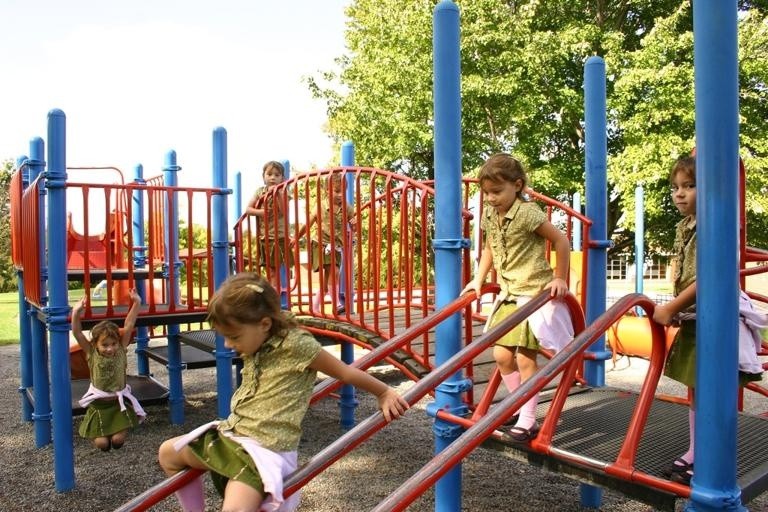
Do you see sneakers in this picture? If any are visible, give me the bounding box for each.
[332,304,346,315]
[501,413,540,443]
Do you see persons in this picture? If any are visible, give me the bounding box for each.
[158,272,410,512]
[459,153,574,444]
[246,160,295,290]
[651,155,767,484]
[71,287,147,451]
[289,173,357,315]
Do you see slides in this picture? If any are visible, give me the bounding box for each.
[67,209,184,380]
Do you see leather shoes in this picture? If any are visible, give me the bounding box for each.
[672,456,694,485]
